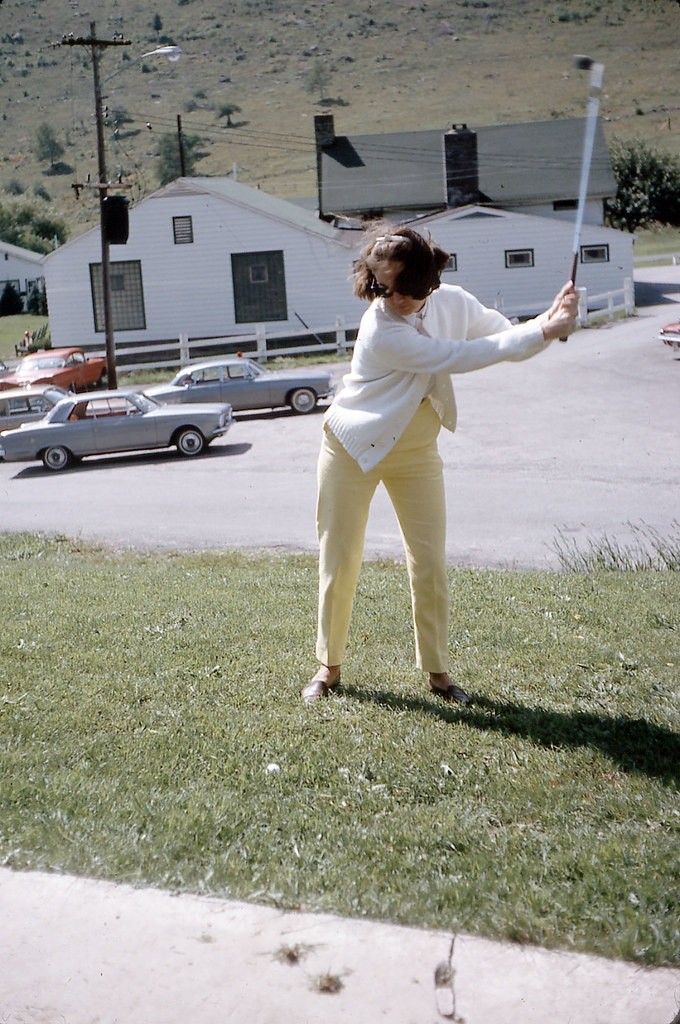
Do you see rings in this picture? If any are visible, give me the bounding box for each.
[574,311,579,317]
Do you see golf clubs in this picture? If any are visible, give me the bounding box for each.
[558,52,608,343]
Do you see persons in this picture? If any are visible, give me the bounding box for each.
[23,331,33,348]
[301,228,581,702]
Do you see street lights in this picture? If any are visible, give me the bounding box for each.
[93,43,185,391]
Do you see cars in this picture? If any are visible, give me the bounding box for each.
[0,390,236,472]
[140,357,334,415]
[0,346,111,393]
[0,382,73,428]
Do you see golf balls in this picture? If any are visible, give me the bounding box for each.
[265,763,282,777]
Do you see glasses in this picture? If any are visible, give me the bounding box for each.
[371,277,413,298]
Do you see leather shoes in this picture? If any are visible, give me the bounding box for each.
[300,672,341,703]
[428,675,471,705]
